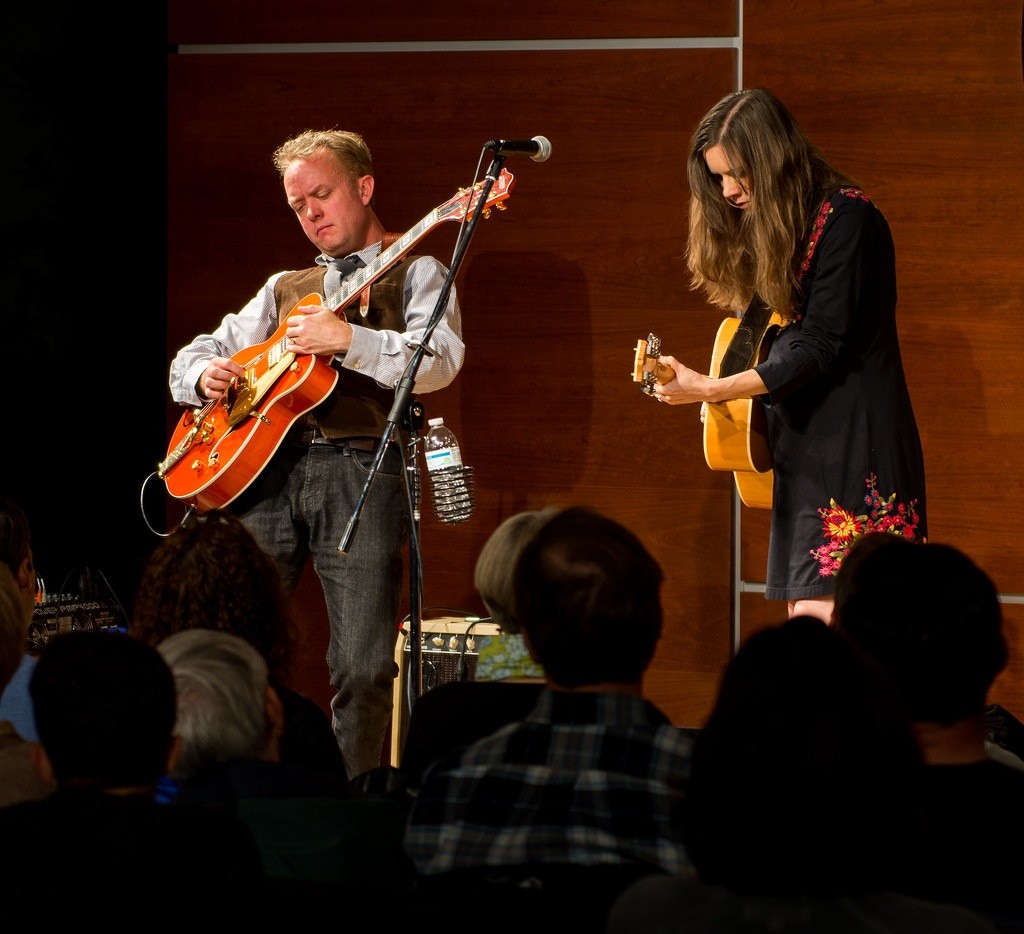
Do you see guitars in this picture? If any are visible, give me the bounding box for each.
[162,163,517,512]
[628,311,795,510]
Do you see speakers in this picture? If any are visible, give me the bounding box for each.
[386,617,505,768]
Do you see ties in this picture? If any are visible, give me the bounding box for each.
[323,254,368,299]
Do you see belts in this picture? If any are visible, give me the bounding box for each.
[281,423,375,452]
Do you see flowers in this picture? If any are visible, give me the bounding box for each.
[808,471,928,578]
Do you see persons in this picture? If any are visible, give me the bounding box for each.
[831,531,1024,933]
[610,612,995,934]
[385,506,687,931]
[165,131,467,779]
[639,88,926,626]
[0,494,349,934]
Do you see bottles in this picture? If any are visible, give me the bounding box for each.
[424,417,472,525]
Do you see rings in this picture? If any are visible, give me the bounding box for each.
[293,338,296,344]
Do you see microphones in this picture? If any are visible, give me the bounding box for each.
[484,135,551,162]
